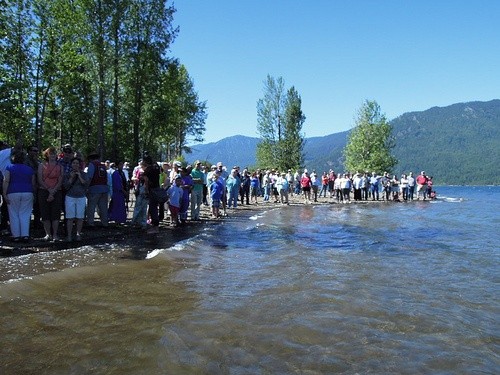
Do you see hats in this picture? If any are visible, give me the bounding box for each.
[194,160,201,166]
[88,150,99,157]
[173,161,181,168]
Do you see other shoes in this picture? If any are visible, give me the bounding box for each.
[13,234,82,243]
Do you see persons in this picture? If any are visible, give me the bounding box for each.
[63,157,92,242]
[37,146,64,242]
[3,150,38,240]
[0,140,442,233]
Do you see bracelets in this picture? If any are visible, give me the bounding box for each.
[3,192,6,195]
[46,186,49,190]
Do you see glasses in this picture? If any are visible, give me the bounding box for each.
[31,149,39,153]
[64,150,71,153]
